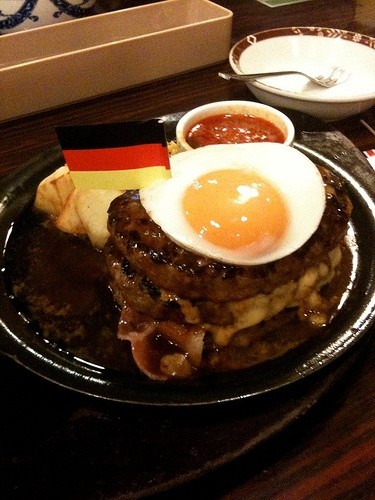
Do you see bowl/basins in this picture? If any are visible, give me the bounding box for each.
[176,101,294,151]
[229,26,375,121]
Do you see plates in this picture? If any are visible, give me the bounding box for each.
[0,113,375,406]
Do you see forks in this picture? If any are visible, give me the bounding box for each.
[218,65,349,87]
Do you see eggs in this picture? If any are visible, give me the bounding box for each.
[140,144,325,263]
